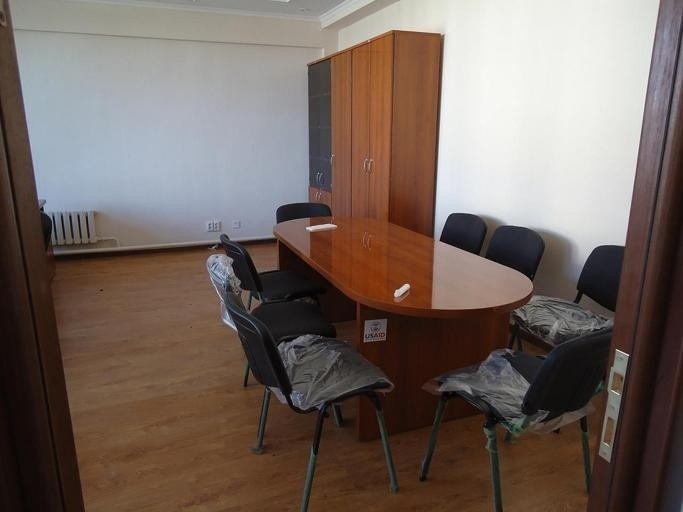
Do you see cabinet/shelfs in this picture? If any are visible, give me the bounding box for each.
[309,29,442,239]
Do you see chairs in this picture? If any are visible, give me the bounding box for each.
[439,212,488,257]
[511,243,624,352]
[275,203,333,223]
[419,325,615,512]
[224,291,401,512]
[206,246,337,392]
[218,232,321,312]
[485,225,546,349]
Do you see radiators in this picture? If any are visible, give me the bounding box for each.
[44,208,98,246]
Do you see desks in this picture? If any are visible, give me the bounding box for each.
[272,216,537,444]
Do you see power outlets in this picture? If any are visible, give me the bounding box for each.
[232,219,240,229]
[208,220,213,231]
[214,221,220,231]
